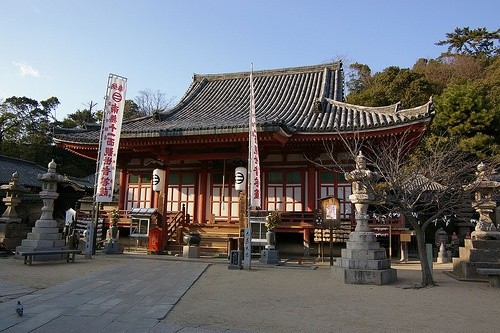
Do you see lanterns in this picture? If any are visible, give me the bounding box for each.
[152,168,166,191]
[235,167,247,191]
